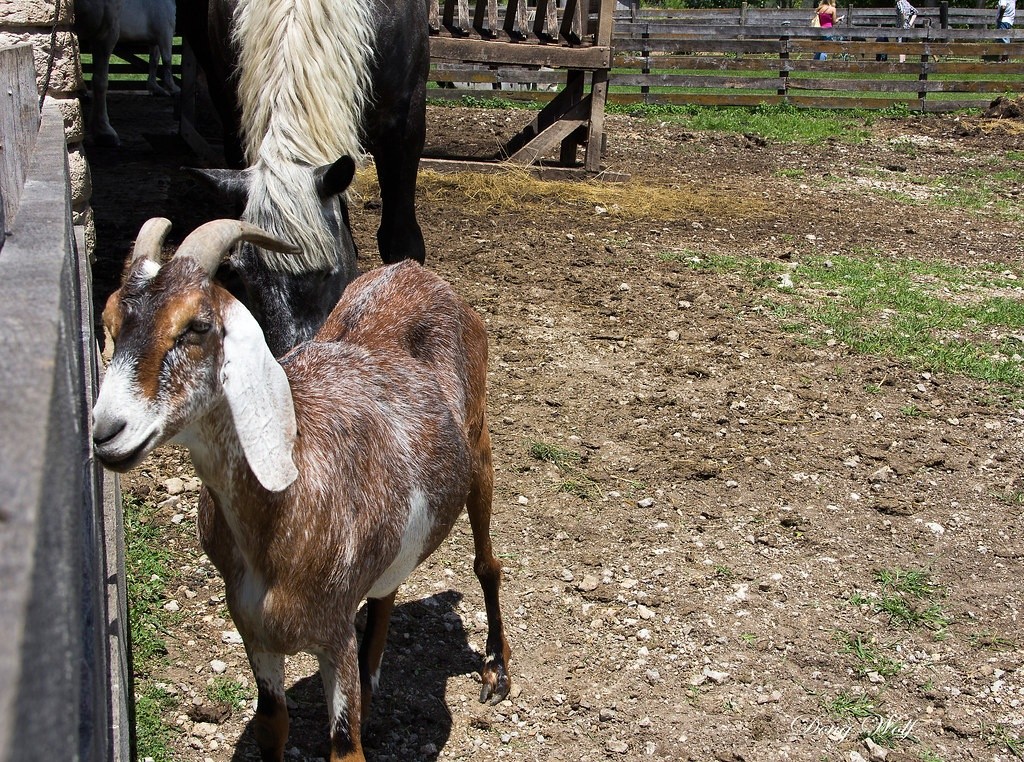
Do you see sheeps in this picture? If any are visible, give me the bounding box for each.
[88,215,514,762]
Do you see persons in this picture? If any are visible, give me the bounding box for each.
[996,0,1015,61]
[895,0,918,63]
[813,0,844,61]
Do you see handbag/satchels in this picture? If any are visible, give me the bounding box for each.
[808,11,821,27]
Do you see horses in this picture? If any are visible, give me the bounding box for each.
[71,0,431,359]
